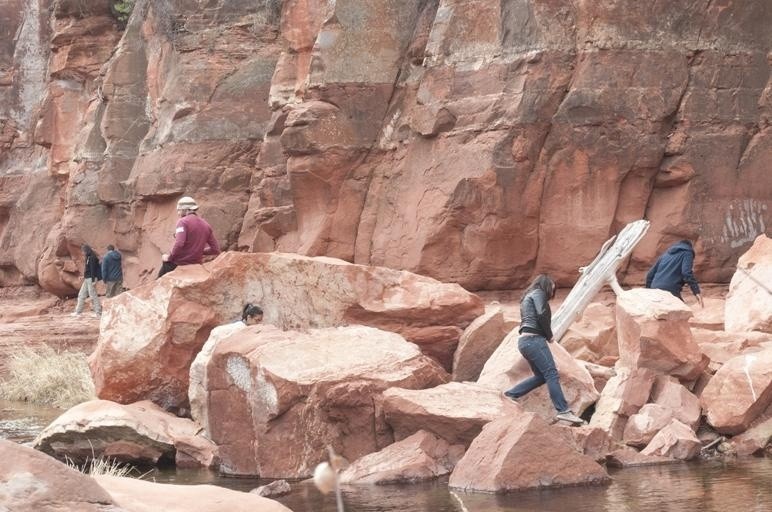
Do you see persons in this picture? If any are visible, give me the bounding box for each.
[233,301,264,326]
[73,245,104,316]
[100,244,123,297]
[646,240,704,309]
[158,197,222,279]
[505,272,585,424]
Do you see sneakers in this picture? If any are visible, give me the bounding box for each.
[557,412,583,423]
[503,392,521,405]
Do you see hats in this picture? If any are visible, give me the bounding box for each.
[177,197,198,210]
